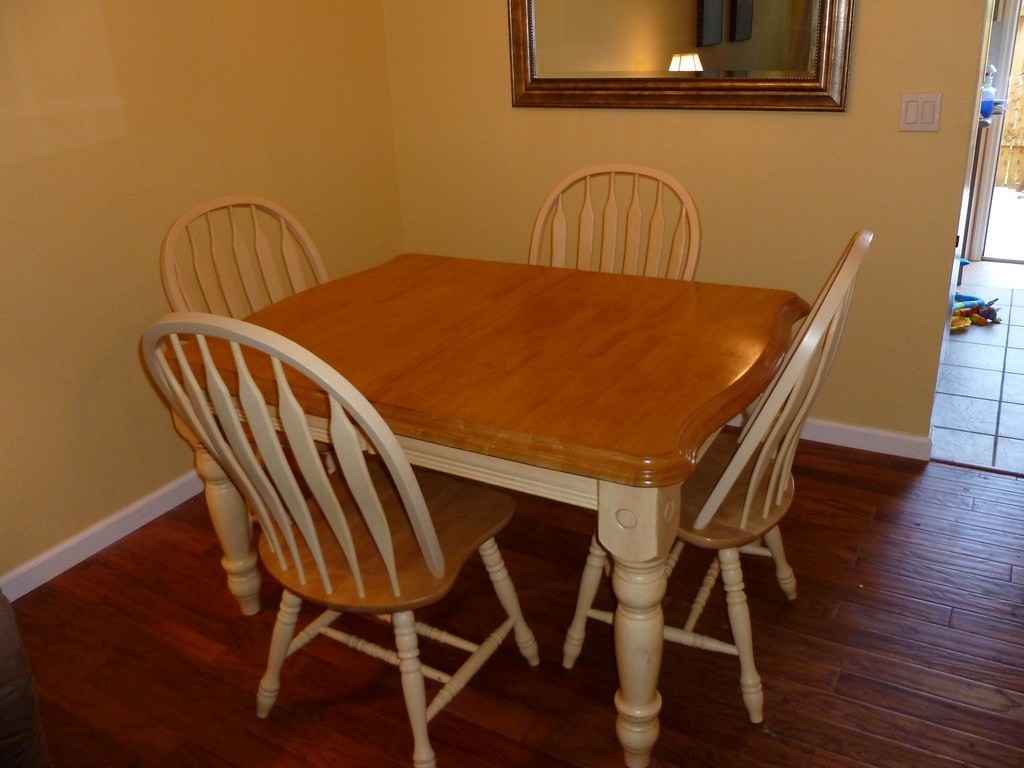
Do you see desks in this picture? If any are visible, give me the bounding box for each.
[154,254,813,768]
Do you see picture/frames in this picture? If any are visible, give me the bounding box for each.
[730,0,753,41]
[697,0,724,46]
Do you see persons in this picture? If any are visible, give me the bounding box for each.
[0,589,54,768]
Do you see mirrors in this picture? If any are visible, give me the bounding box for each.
[507,0,855,111]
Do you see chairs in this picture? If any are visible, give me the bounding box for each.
[560,229,873,725]
[530,164,702,283]
[162,197,336,533]
[139,310,541,768]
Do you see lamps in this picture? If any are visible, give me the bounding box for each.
[669,53,703,72]
